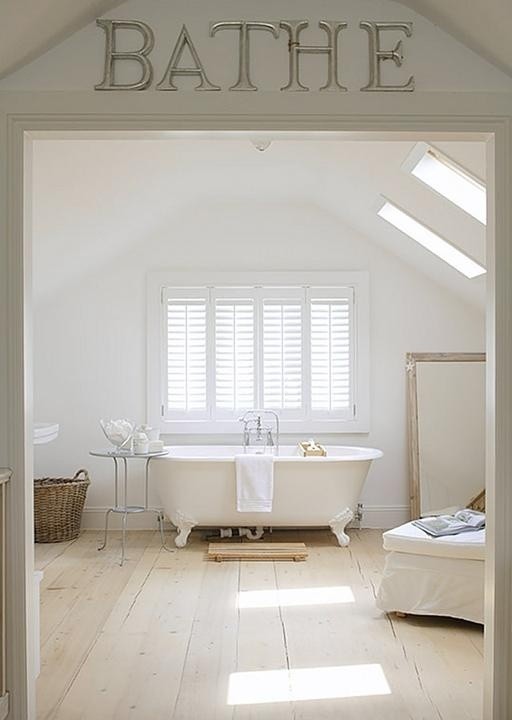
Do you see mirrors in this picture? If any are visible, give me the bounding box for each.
[406,352,486,522]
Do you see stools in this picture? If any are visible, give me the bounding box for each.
[377,516,486,627]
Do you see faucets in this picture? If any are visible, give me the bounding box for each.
[256,434,264,442]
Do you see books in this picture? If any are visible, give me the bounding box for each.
[411,509,486,537]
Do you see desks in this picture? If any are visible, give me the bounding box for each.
[89,448,173,567]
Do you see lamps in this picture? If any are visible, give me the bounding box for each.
[250,138,271,152]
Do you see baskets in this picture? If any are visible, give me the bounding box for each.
[34,469,91,543]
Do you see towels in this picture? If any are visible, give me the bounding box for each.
[235,453,274,513]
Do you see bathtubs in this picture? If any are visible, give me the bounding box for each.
[148,445,385,548]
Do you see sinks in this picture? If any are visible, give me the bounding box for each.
[33,423,60,445]
